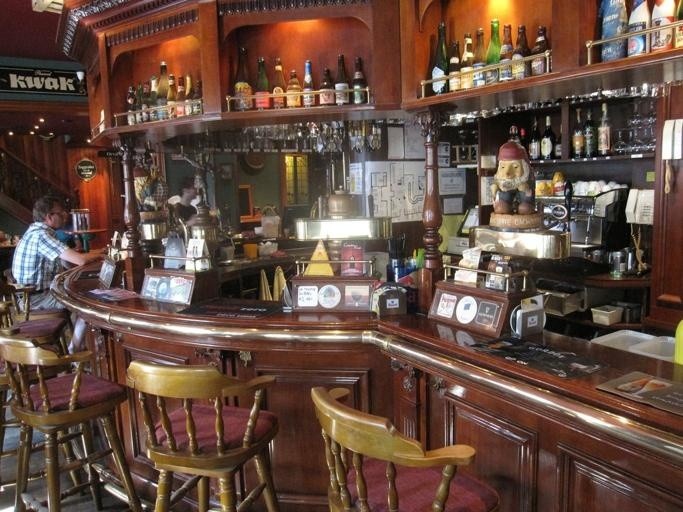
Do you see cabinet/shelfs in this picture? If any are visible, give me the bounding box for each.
[89,0,399,147]
[477,90,657,169]
[546,268,646,334]
[400,1,683,111]
[220,275,258,298]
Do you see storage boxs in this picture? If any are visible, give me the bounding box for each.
[590,330,657,352]
[591,304,624,326]
[629,336,675,361]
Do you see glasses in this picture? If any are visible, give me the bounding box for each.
[49,211,64,216]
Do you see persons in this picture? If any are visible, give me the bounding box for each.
[11,197,107,310]
[492,143,535,214]
[175,175,198,225]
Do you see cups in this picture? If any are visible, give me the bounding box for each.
[218,124,296,150]
[219,246,234,260]
[243,244,258,259]
[611,128,628,154]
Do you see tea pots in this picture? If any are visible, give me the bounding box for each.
[328,185,356,216]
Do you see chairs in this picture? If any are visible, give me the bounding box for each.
[124,358,280,511]
[5,268,17,286]
[312,387,501,510]
[1,336,142,510]
[0,302,67,355]
[0,289,69,355]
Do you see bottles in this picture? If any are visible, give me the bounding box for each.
[627,99,657,151]
[126,60,202,126]
[429,19,550,93]
[232,47,254,110]
[287,70,301,107]
[253,56,272,109]
[334,54,350,104]
[507,102,611,160]
[319,67,335,105]
[271,56,287,108]
[351,56,367,104]
[302,60,315,106]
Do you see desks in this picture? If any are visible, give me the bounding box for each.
[64,229,106,252]
